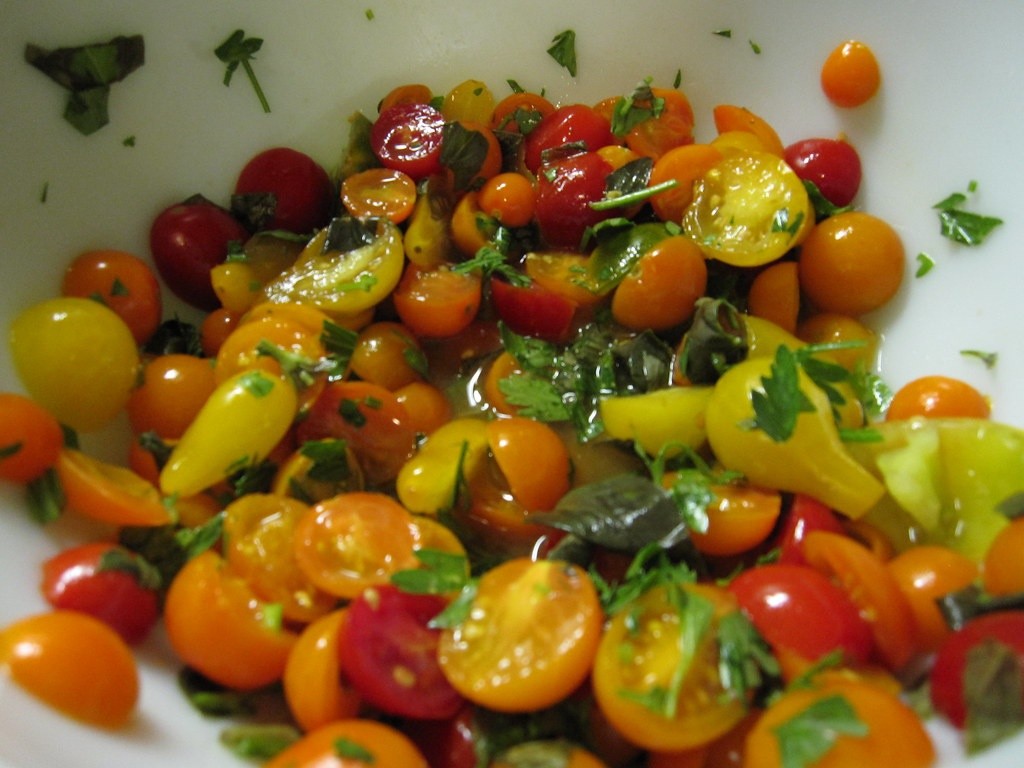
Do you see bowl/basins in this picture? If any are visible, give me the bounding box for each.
[0,0,1024,768]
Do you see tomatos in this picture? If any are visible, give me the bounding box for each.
[0,40,1024,768]
